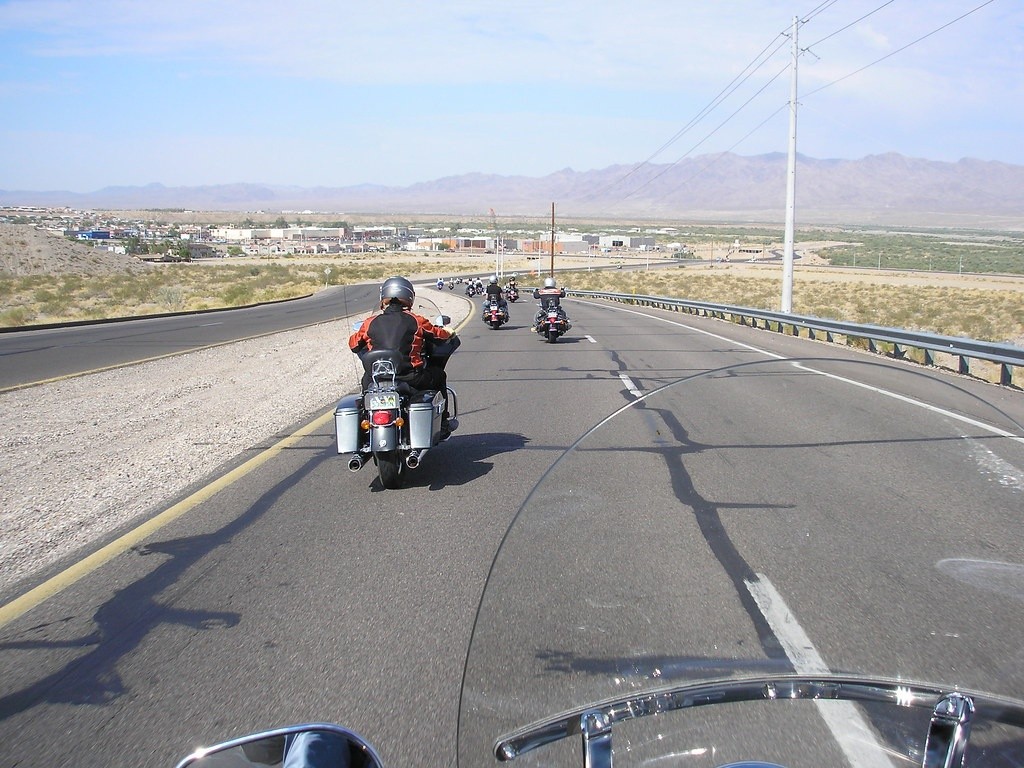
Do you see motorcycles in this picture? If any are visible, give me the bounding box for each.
[474,282,484,295]
[447,281,455,290]
[482,293,508,330]
[533,288,572,344]
[465,283,476,298]
[456,279,462,285]
[437,281,444,291]
[505,285,520,303]
[334,313,459,490]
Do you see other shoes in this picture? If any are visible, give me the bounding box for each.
[566,324,572,330]
[440,419,459,438]
[531,327,538,333]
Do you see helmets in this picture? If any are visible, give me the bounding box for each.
[379,275,416,308]
[489,275,499,284]
[469,277,472,281]
[477,276,480,280]
[544,277,557,288]
[511,277,516,281]
[509,278,513,281]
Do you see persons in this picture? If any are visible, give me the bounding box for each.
[349,276,456,440]
[437,276,570,331]
